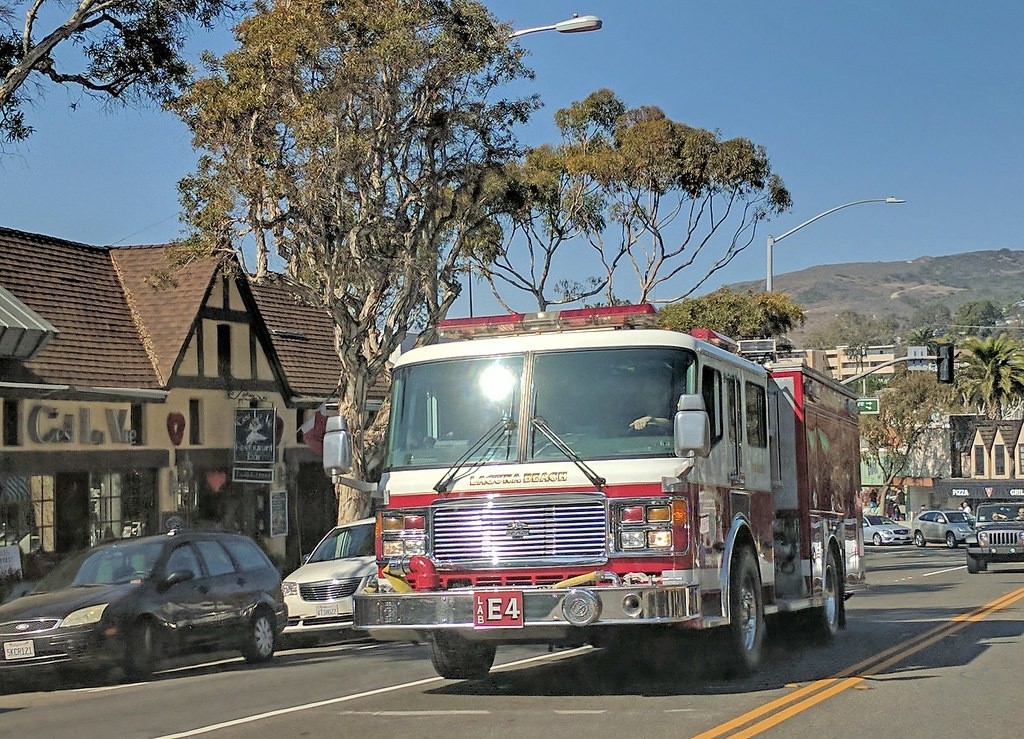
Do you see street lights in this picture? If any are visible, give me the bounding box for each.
[399,14,603,201]
[764,196,906,293]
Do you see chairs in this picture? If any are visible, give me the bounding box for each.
[113,566,137,585]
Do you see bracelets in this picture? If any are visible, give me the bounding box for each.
[652,416,655,422]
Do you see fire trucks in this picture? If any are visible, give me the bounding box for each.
[321,302,866,686]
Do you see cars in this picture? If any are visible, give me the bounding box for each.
[862,514,914,546]
[0,528,290,687]
[279,515,381,647]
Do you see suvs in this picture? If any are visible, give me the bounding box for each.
[967,501,1024,574]
[911,508,977,549]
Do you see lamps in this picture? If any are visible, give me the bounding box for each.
[49,408,60,419]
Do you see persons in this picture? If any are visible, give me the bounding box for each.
[1013,507,1024,520]
[629,382,673,430]
[165,517,182,530]
[991,512,1007,520]
[918,504,927,514]
[959,502,971,521]
[886,498,901,521]
[869,489,878,508]
